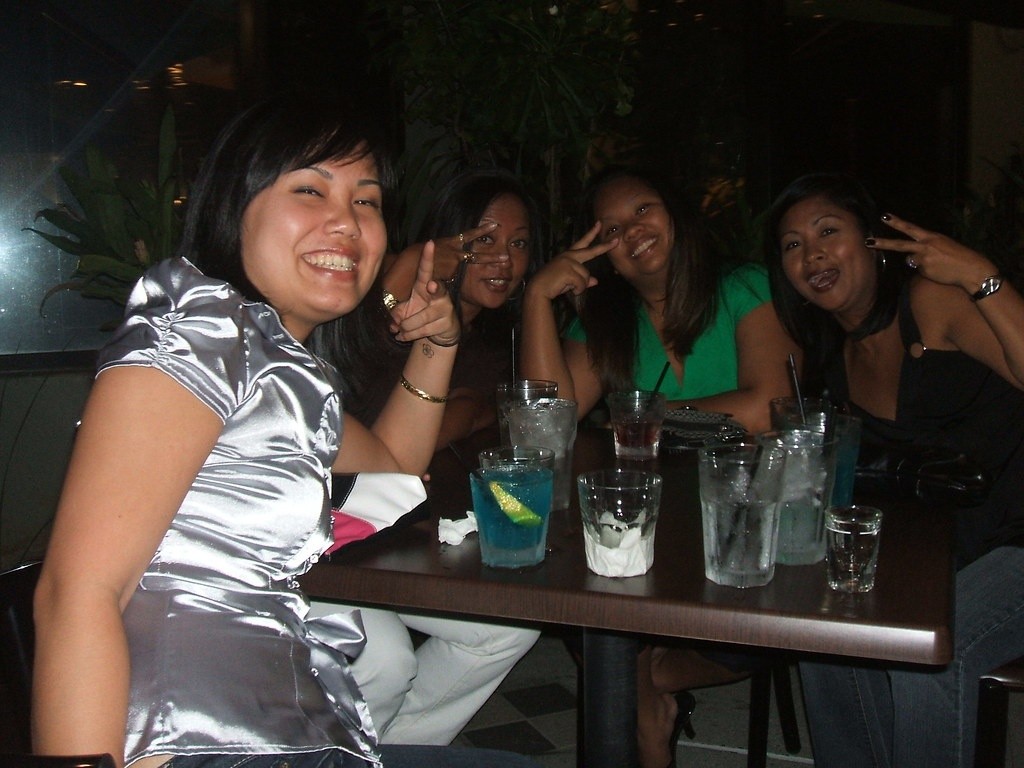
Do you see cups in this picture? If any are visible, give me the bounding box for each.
[479,446,554,474]
[758,432,838,565]
[496,380,558,447]
[470,465,554,569]
[825,506,882,594]
[698,446,788,587]
[828,414,863,507]
[605,391,666,462]
[577,471,662,578]
[509,398,579,511]
[769,397,838,443]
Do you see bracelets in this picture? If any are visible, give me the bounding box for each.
[383,291,398,308]
[428,336,459,347]
[399,373,449,403]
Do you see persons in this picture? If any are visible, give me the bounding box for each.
[28,85,539,768]
[760,172,1024,768]
[520,162,803,768]
[330,168,542,747]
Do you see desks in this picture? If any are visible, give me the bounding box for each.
[297,421,952,767]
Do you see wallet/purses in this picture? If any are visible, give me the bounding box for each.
[660,405,747,449]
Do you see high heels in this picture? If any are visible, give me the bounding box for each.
[639,691,697,768]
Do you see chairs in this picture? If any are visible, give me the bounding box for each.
[0,559,121,768]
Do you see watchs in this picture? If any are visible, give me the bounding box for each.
[970,270,1005,302]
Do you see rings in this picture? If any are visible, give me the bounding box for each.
[906,255,918,268]
[464,249,474,264]
[459,233,464,243]
